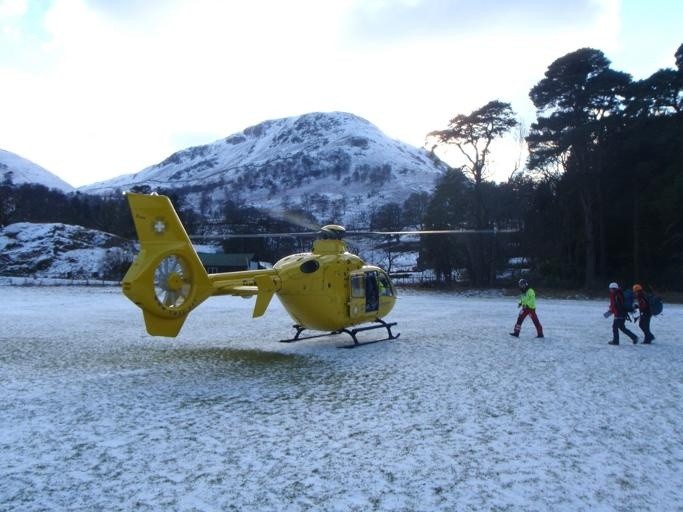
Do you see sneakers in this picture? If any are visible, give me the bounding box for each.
[510,332,519,337]
[535,335,543,338]
[634,336,638,344]
[643,334,654,344]
[609,341,618,345]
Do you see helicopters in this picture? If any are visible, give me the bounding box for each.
[123,188,526,350]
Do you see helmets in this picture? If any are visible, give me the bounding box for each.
[609,283,618,288]
[518,279,529,288]
[633,285,641,293]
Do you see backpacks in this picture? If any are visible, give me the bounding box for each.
[615,290,634,311]
[642,292,662,315]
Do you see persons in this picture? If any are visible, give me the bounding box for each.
[603,282,639,346]
[509,278,544,339]
[633,284,656,345]
